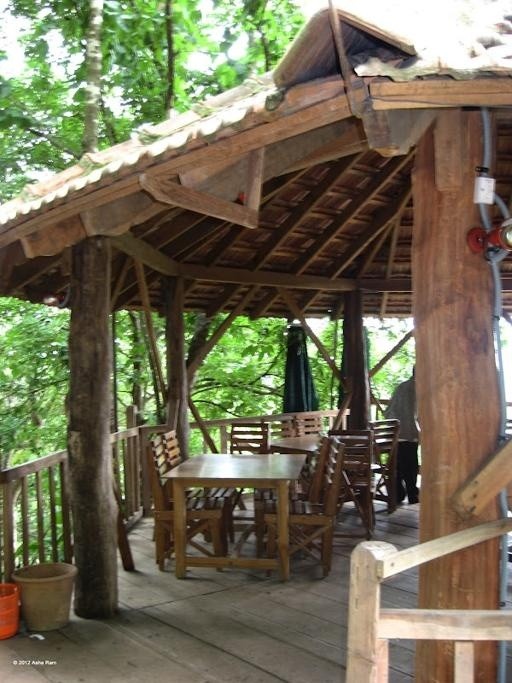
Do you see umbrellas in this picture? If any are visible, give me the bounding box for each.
[279,323,324,437]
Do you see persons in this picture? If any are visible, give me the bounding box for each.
[382,362,419,507]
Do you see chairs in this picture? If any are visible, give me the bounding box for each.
[145,413,402,581]
